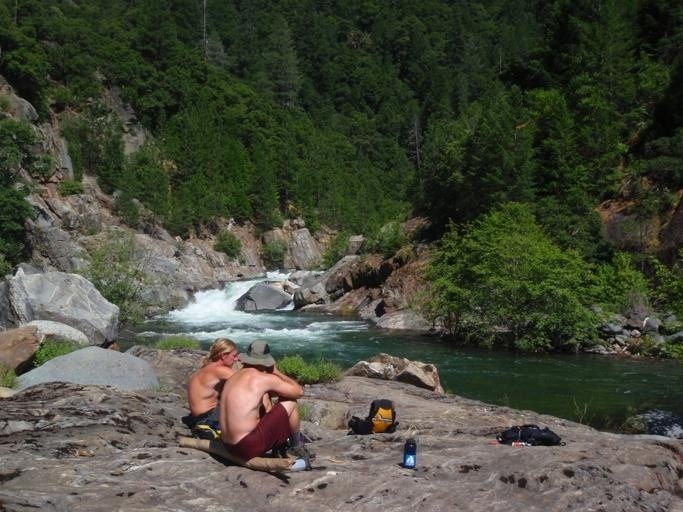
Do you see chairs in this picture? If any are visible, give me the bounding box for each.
[285,445,315,461]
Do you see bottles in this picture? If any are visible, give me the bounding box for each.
[237,340,275,367]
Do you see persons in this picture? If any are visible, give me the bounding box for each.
[218,339,316,464]
[187,339,274,435]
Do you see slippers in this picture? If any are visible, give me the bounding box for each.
[364,399,398,433]
[495,425,565,446]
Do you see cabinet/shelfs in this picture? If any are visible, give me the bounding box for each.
[512,442,531,447]
[386,421,399,433]
[403,438,417,468]
